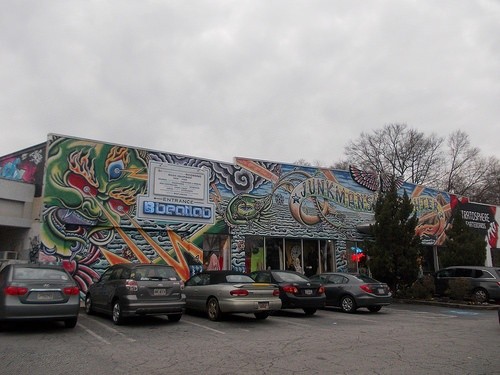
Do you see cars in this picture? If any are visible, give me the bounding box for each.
[0,264,80,329]
[184,270,282,322]
[309,272,392,313]
[249,269,326,315]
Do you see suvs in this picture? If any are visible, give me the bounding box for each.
[84,264,184,325]
[430,265,500,304]
